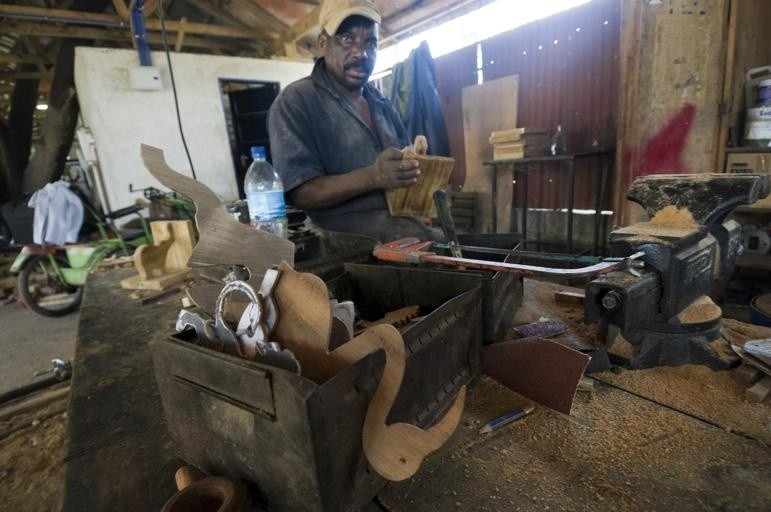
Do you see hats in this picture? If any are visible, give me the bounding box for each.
[319,0,382,39]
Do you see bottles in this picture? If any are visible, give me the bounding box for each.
[549,124,568,156]
[244,146,288,241]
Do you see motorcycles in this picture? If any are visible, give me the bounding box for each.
[7,183,197,316]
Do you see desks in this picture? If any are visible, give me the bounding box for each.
[483,151,609,258]
[61,262,771,511]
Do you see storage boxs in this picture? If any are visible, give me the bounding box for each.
[149,232,525,512]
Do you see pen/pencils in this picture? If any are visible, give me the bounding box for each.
[477,403,536,434]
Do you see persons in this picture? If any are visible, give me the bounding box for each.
[265,0,448,267]
